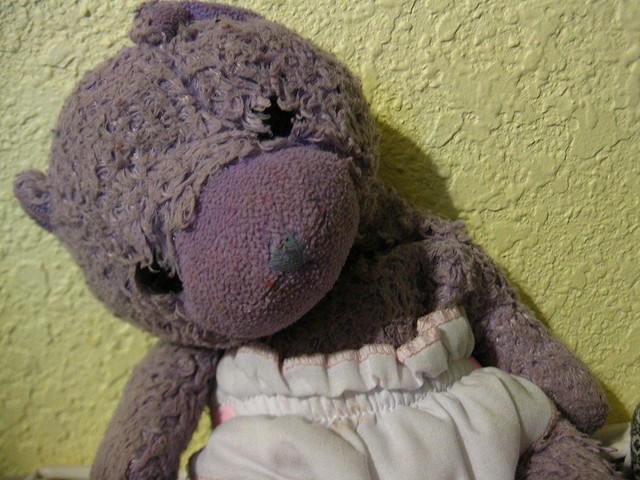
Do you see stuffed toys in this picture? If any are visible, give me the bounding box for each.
[12,1,624,480]
